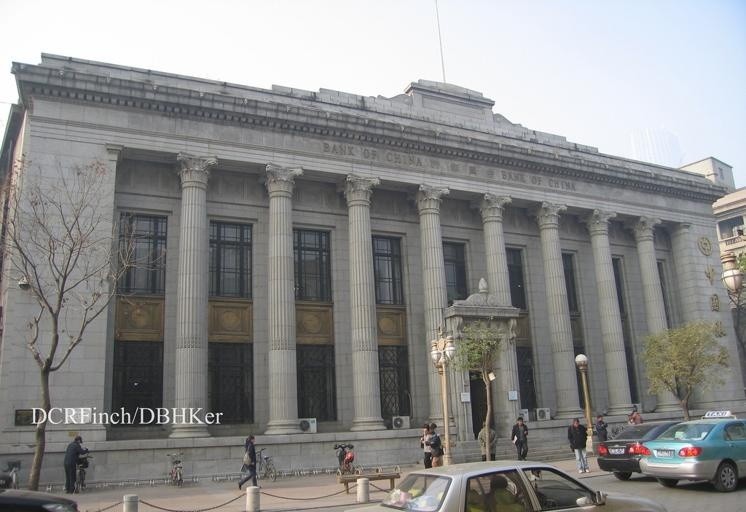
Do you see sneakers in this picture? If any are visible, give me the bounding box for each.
[579,470,583,473]
[585,469,589,473]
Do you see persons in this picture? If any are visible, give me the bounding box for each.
[595,415,608,441]
[510,416,529,461]
[486,475,508,512]
[426,423,444,467]
[237,434,261,490]
[478,420,497,460]
[63,435,88,493]
[420,424,432,468]
[567,418,590,473]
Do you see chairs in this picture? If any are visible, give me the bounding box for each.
[467,488,525,511]
[675,430,708,440]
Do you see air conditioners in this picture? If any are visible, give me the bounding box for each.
[533,407,551,420]
[299,417,317,433]
[392,415,411,429]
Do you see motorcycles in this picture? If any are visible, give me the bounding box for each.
[334,442,354,476]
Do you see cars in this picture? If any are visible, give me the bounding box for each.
[638,410,746,492]
[0,483,79,512]
[596,419,681,481]
[342,459,669,512]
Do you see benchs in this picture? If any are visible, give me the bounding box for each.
[337,472,400,494]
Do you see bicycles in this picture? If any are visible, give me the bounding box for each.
[4,459,22,488]
[74,453,94,492]
[254,447,277,481]
[168,451,185,486]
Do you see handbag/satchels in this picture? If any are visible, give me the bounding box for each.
[243,450,254,467]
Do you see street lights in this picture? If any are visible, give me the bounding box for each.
[721,249,746,293]
[430,325,459,465]
[575,353,595,429]
